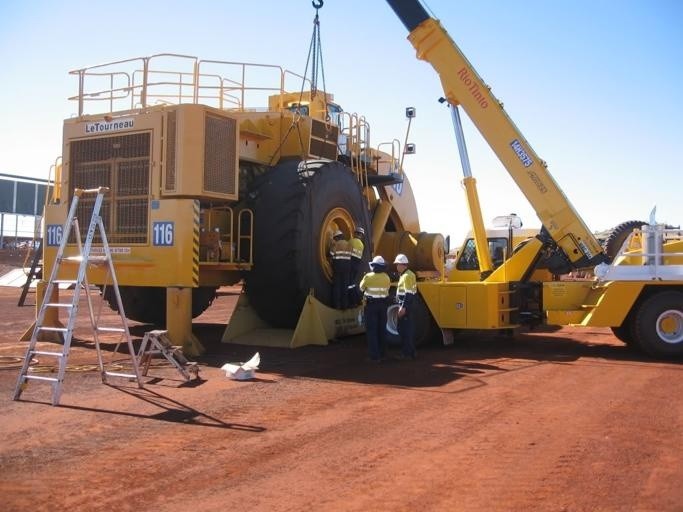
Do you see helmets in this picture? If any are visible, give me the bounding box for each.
[394,254,408,264]
[354,227,364,234]
[333,231,344,238]
[370,255,386,266]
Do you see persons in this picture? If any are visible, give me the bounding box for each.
[393,253,417,363]
[348,227,366,309]
[329,230,353,310]
[358,255,391,367]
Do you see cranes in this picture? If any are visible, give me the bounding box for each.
[310,1,683,361]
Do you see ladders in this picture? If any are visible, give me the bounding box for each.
[13,184,144,408]
[131,328,202,383]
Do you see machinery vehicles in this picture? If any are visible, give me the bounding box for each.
[24,53,451,359]
